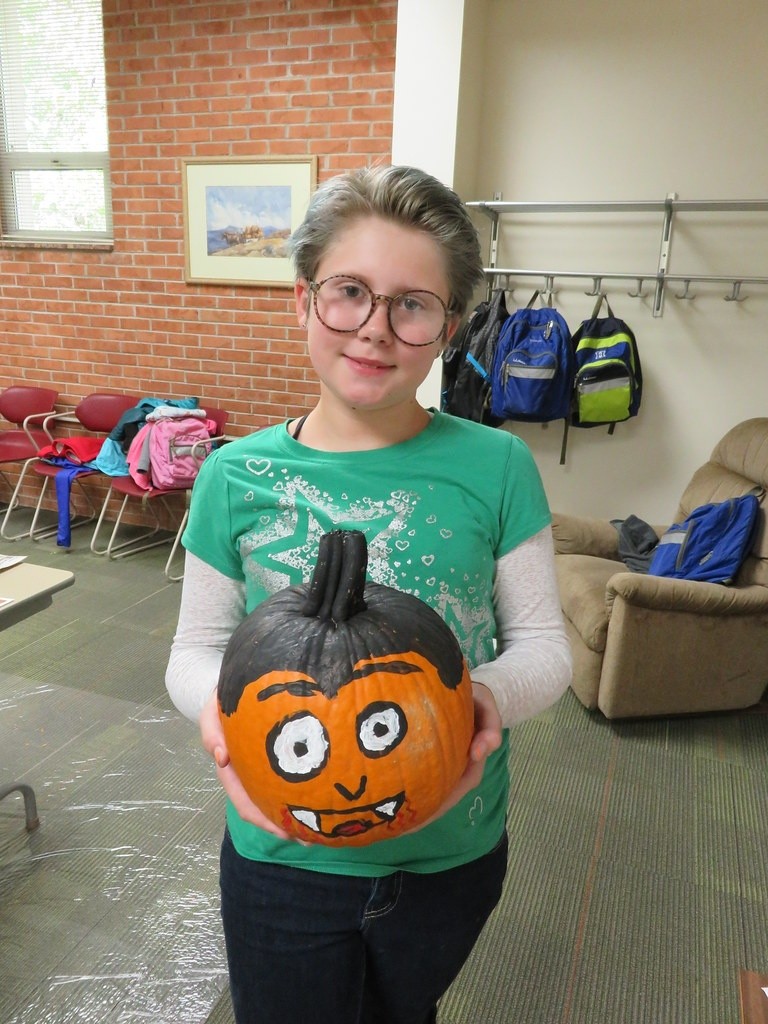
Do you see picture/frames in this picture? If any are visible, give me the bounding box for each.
[181,152,319,289]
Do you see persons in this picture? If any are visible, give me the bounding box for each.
[163,166,573,1024]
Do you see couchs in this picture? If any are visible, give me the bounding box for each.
[551,417,768,721]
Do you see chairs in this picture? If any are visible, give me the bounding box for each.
[0,385,230,580]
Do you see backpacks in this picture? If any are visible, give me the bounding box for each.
[609,486,766,584]
[564,293,643,429]
[442,289,513,429]
[148,414,211,493]
[490,289,574,423]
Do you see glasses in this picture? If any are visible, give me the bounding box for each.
[305,274,457,346]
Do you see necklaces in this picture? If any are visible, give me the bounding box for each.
[291,412,309,440]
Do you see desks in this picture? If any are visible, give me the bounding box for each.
[0,562,75,830]
